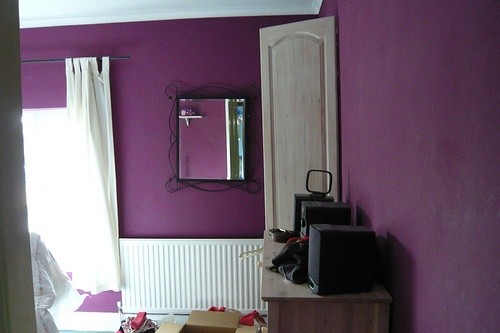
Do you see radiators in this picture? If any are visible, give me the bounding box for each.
[115,237,270,318]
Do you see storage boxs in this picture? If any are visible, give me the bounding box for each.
[154,308,256,333]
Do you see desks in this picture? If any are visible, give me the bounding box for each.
[260,229,393,332]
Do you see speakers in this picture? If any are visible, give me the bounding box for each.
[294,192,375,296]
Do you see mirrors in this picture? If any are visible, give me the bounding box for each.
[177,95,246,181]
